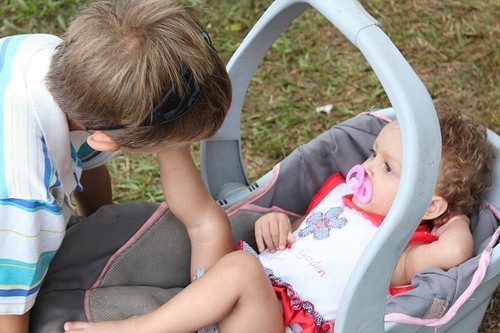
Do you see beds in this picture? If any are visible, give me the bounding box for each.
[27,0,500,332]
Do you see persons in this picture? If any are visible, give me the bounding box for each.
[0,0,234,333]
[59,100,496,333]
